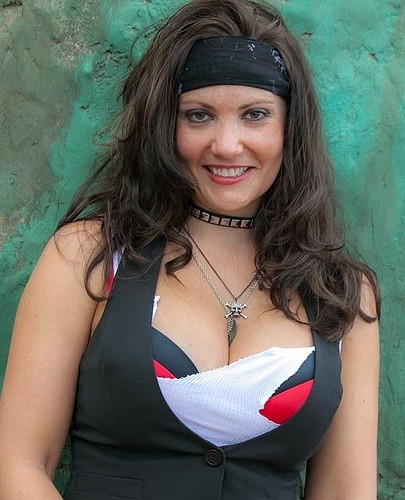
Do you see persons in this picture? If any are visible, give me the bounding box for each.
[0,1,382,500]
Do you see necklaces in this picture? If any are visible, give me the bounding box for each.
[177,225,267,346]
[185,203,257,229]
